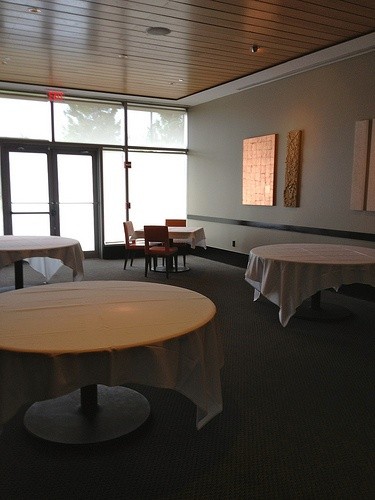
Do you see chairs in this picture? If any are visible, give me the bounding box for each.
[162,220,186,267]
[123,221,153,271]
[144,225,178,279]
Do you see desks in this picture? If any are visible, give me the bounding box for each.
[0,281,223,444]
[130,226,206,273]
[244,244,375,327]
[0,236,84,290]
